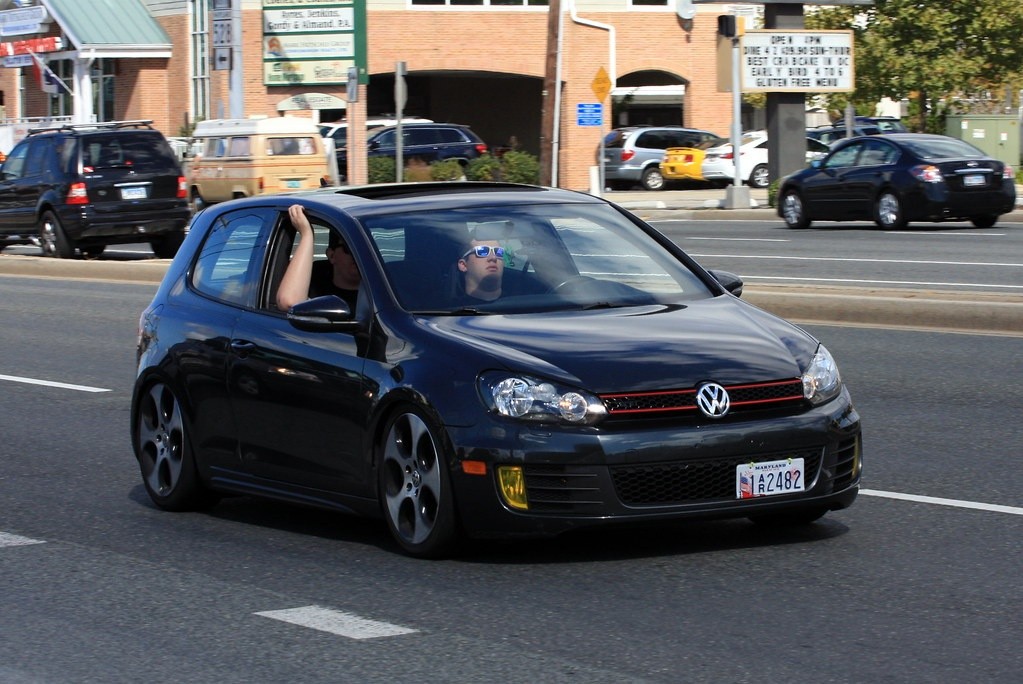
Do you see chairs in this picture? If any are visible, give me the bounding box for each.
[306,258,340,302]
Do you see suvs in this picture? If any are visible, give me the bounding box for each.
[2,120,200,260]
[319,117,486,182]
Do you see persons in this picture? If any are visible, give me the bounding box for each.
[275,205,360,321]
[437,229,519,311]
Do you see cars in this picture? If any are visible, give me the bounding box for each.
[132,182,863,550]
[596,117,907,191]
[777,134,1017,226]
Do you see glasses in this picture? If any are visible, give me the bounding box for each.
[463,245,505,259]
[334,241,352,254]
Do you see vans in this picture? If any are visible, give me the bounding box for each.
[182,116,332,206]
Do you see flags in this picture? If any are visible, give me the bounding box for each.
[32,55,68,94]
